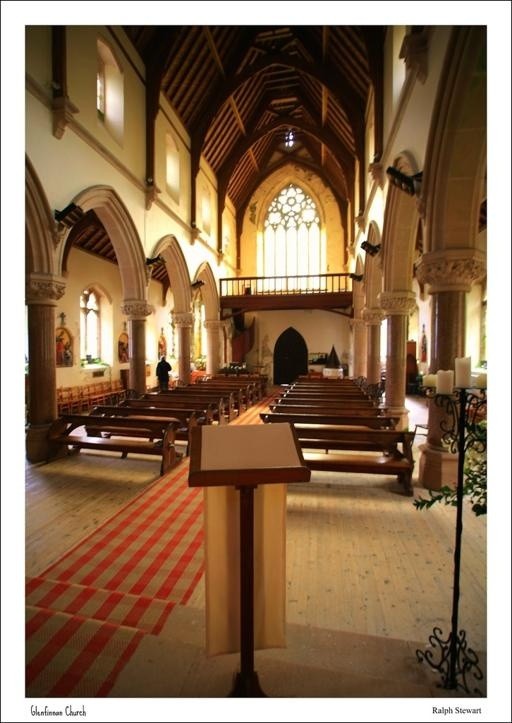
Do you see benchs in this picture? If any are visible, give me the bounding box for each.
[258,372,417,497]
[51,373,268,477]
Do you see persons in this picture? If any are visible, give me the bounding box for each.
[155,354,172,391]
[64,340,73,365]
[118,341,129,363]
[56,329,65,365]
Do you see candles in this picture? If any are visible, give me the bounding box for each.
[422,356,486,396]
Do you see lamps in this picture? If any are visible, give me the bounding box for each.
[385,165,422,198]
[349,273,363,282]
[192,280,205,289]
[146,257,167,269]
[360,241,381,258]
[54,202,86,230]
